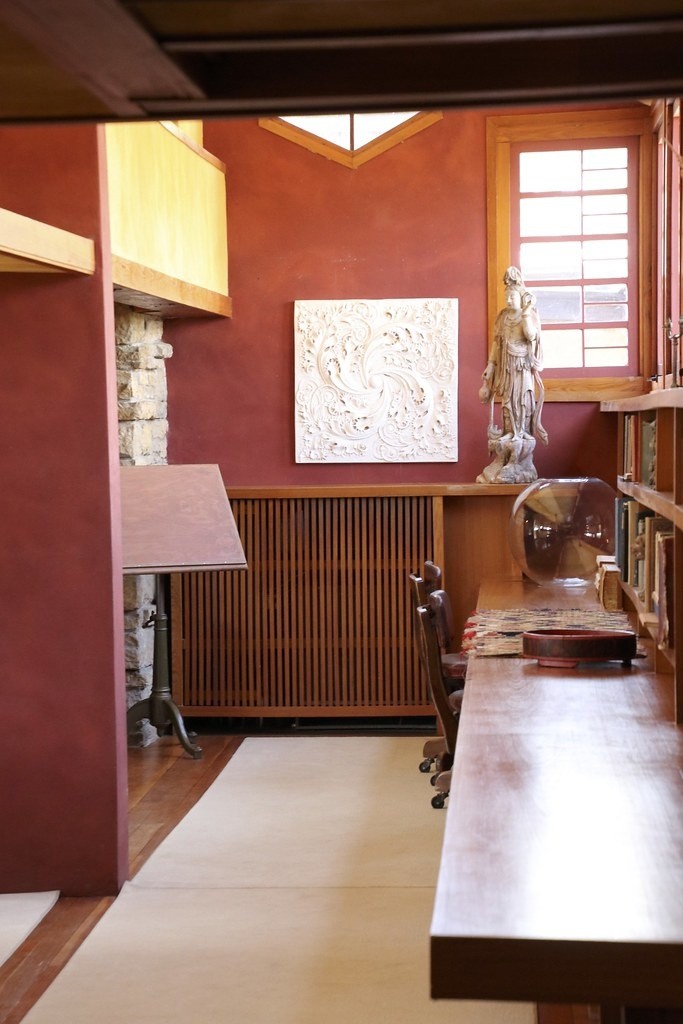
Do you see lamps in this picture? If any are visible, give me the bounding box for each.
[119,464,252,760]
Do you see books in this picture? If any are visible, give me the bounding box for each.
[614,497,674,649]
[624,415,653,482]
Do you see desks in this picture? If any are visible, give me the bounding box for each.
[429,577,683,1024]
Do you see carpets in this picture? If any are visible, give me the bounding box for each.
[0,734,542,1024]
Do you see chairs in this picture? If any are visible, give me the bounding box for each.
[400,560,469,811]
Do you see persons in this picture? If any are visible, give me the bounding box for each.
[481,285,536,441]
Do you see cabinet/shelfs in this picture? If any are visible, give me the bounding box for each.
[104,119,234,324]
[599,94,683,728]
